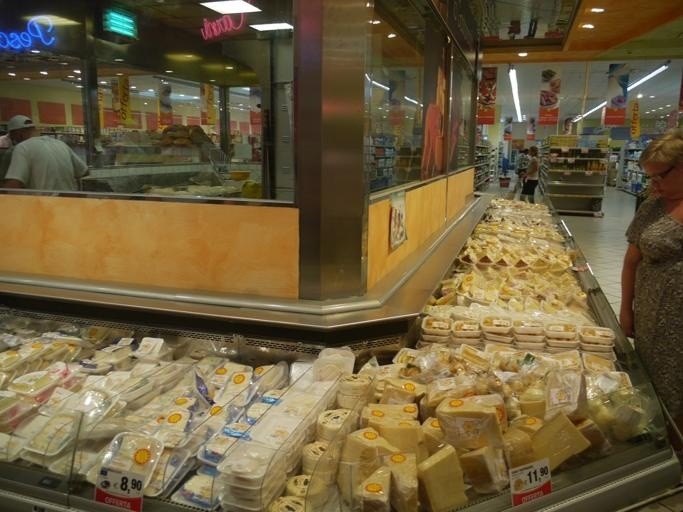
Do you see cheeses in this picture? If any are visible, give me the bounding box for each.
[336,378,646,512]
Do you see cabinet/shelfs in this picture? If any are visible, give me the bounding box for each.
[474,136,654,218]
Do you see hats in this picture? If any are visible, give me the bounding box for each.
[7,114,37,132]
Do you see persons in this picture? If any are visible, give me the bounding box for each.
[501,154,509,177]
[517,144,537,206]
[0,121,229,197]
[516,148,531,190]
[614,124,682,455]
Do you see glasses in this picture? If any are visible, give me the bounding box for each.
[645,167,672,182]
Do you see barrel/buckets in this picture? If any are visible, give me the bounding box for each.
[500,177,511,187]
